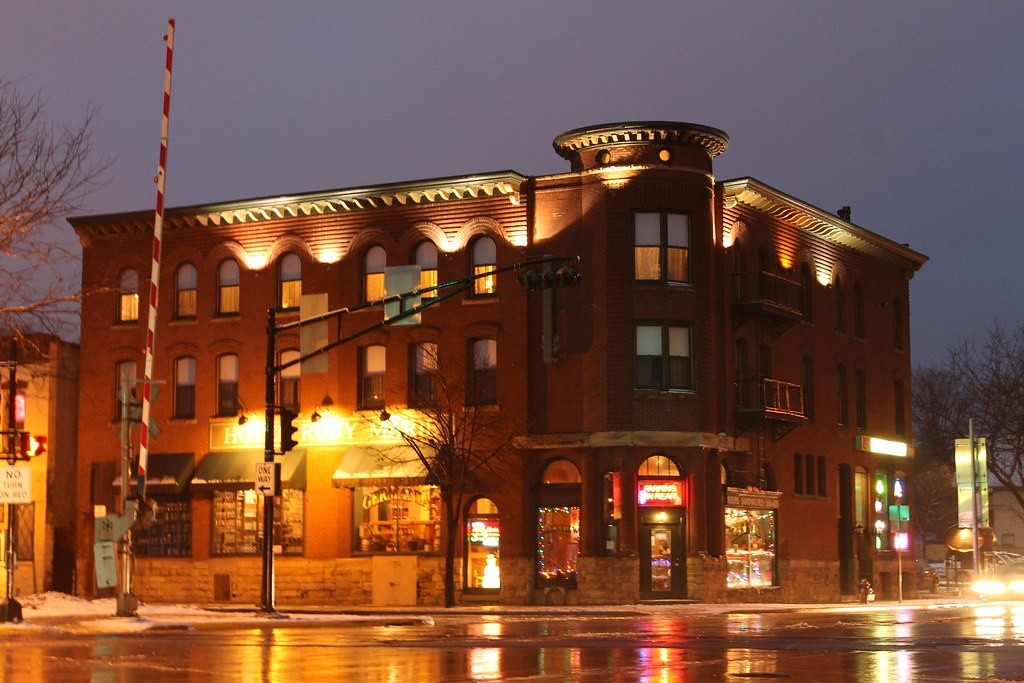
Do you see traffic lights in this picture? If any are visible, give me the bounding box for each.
[277,406,299,452]
[20,432,45,462]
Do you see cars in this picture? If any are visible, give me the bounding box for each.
[915,560,940,594]
[984,564,1024,600]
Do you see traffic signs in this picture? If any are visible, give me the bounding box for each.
[255,462,275,496]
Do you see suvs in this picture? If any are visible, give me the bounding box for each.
[945,552,1018,583]
